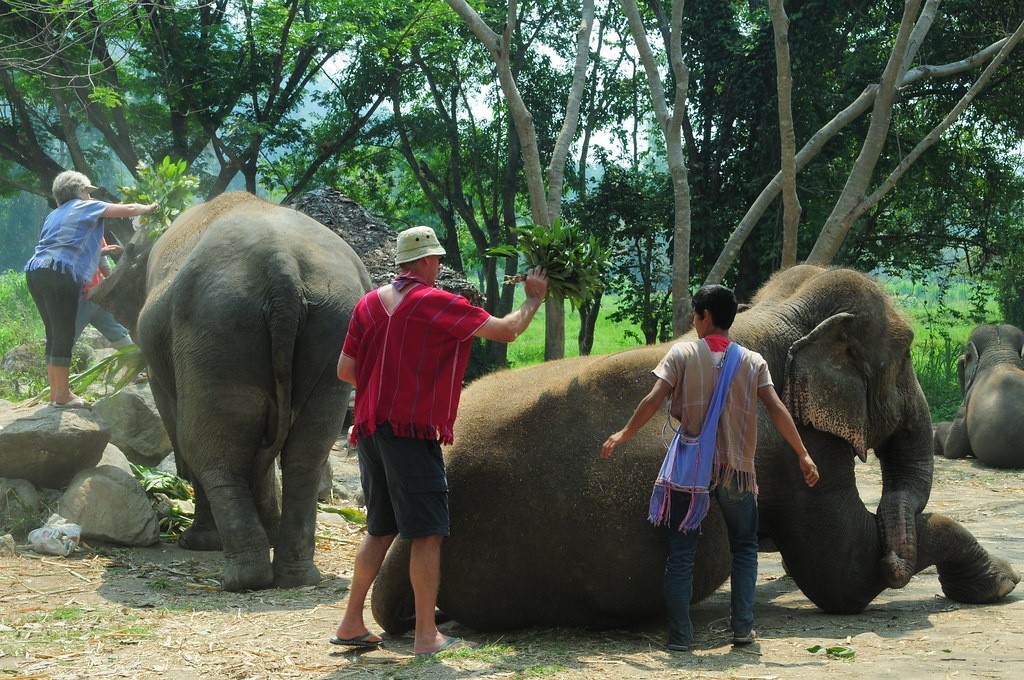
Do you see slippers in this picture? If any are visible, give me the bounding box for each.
[330,630,382,646]
[733,630,757,642]
[668,643,689,651]
[414,637,481,655]
[52,396,93,409]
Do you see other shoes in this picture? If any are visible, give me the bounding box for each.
[134,370,147,384]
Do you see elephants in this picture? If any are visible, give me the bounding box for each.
[85,189,375,591]
[369,261,1024,625]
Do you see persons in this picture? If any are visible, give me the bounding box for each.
[329,226,550,656]
[24,171,158,408]
[600,284,820,651]
[72,235,149,384]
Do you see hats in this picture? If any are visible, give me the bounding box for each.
[394,226,446,265]
[78,179,98,193]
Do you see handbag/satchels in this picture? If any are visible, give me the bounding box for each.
[645,425,718,532]
